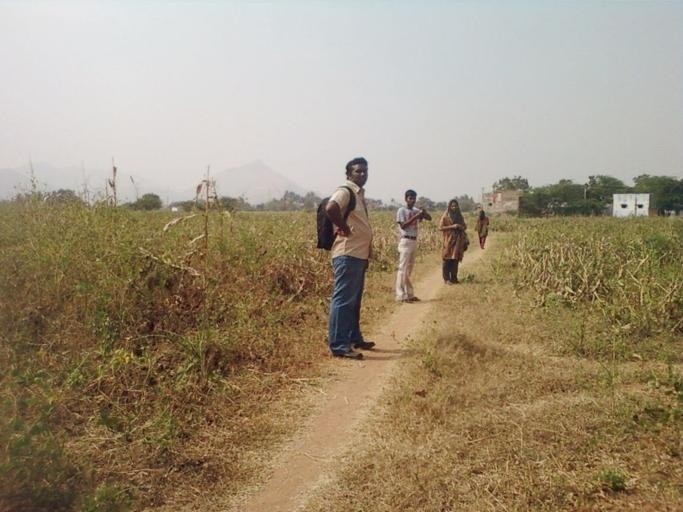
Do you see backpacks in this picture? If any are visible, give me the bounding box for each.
[317,187,355,250]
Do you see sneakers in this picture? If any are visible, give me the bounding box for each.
[352,341,374,349]
[334,350,362,359]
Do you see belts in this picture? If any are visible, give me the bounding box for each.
[404,236,416,239]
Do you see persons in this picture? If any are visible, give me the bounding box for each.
[437,198,469,285]
[326,156,376,359]
[395,189,433,304]
[473,209,489,250]
